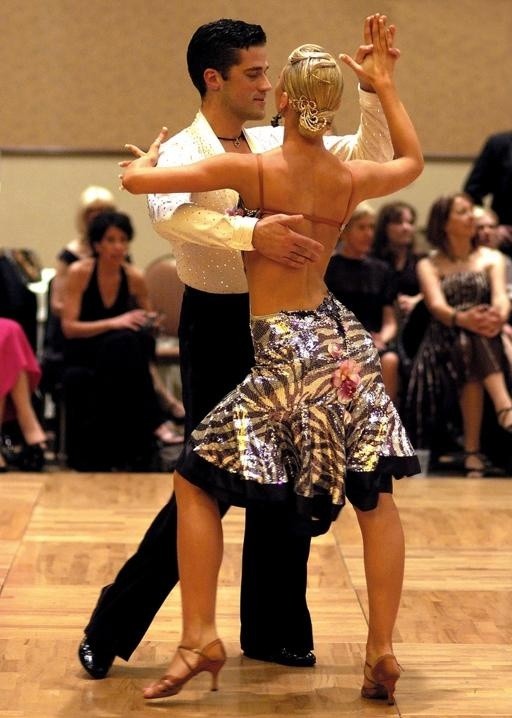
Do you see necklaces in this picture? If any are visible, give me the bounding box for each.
[215,131,247,148]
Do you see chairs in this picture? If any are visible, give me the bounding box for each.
[42,276,156,461]
[145,257,187,365]
[396,299,503,462]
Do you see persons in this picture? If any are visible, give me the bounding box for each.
[327,201,398,409]
[78,10,401,679]
[473,209,502,249]
[405,193,511,472]
[369,204,431,362]
[51,184,185,450]
[1,318,50,471]
[62,209,188,483]
[118,28,424,705]
[463,129,511,231]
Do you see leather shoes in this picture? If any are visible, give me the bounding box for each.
[79,637,114,680]
[244,647,316,666]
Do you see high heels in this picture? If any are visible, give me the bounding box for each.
[22,434,56,463]
[166,400,186,427]
[152,423,185,450]
[461,450,484,479]
[143,640,227,699]
[495,406,512,431]
[363,655,405,705]
